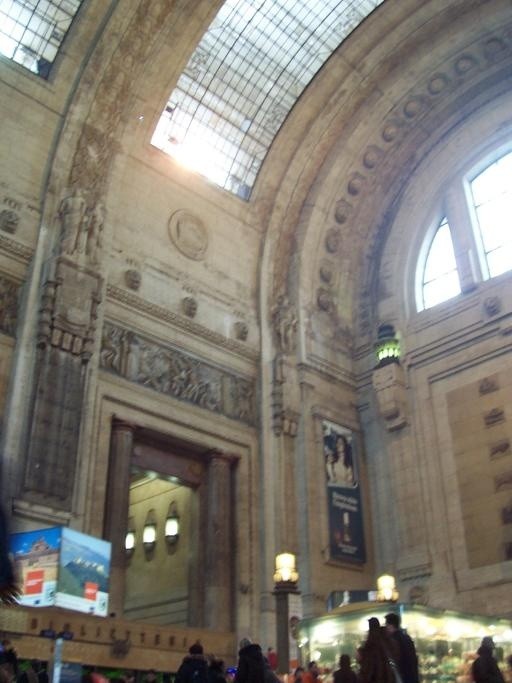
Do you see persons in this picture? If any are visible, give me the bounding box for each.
[2,613,512,682]
[325,435,356,488]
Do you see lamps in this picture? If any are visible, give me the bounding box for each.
[125,520,135,567]
[274,553,299,584]
[373,324,401,367]
[142,510,157,560]
[378,573,398,603]
[165,502,180,554]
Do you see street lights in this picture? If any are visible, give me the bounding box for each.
[375,569,401,620]
[38,628,73,683]
[273,552,303,675]
